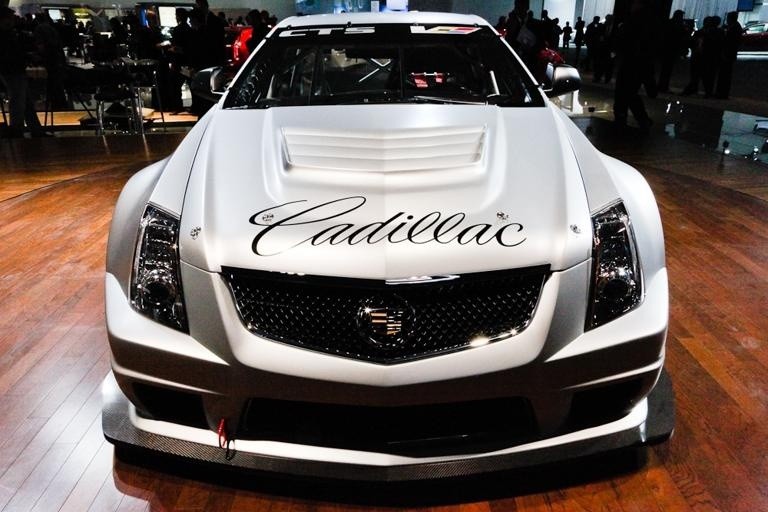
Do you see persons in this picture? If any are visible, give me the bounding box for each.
[607,0,672,136]
[0,0,279,120]
[0,0,54,137]
[488,0,743,101]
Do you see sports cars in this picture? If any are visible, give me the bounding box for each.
[100,11,675,483]
